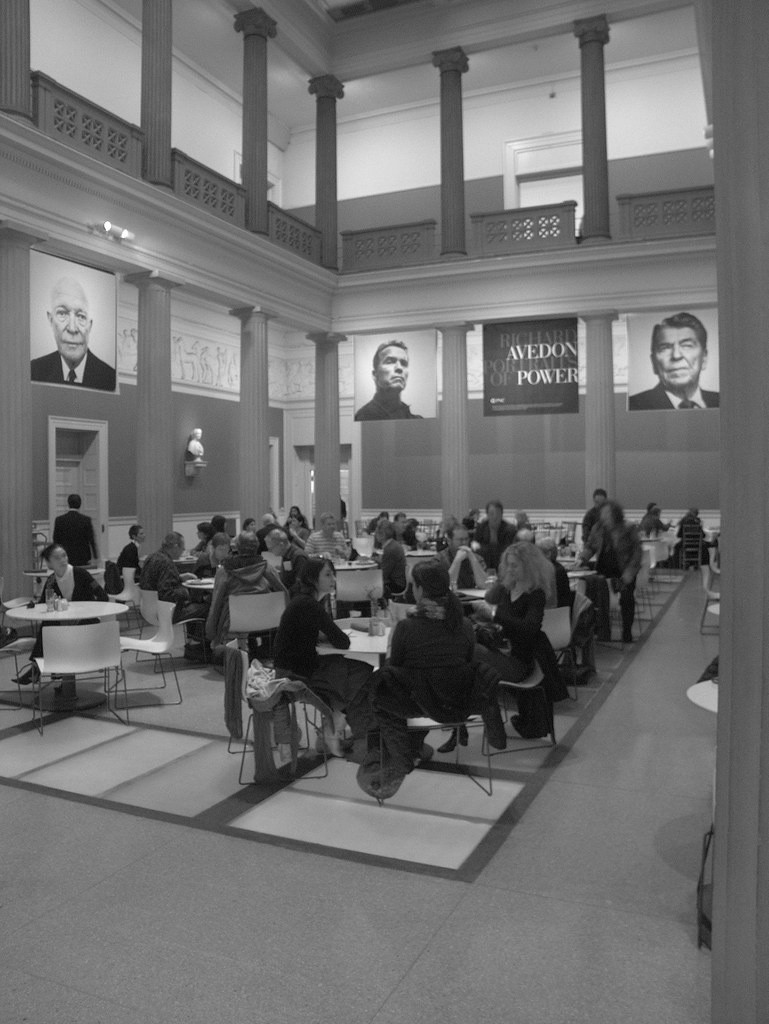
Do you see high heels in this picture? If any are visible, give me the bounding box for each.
[10,667,40,684]
[436,726,468,753]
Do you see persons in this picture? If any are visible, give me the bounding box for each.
[30,277,117,390]
[628,313,720,410]
[355,339,422,420]
[188,428,204,455]
[12,486,711,763]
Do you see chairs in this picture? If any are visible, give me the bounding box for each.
[0,521,723,809]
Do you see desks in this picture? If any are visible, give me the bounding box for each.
[183,577,214,591]
[687,679,718,943]
[6,602,129,711]
[568,569,604,577]
[318,629,387,664]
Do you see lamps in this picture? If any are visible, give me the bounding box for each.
[91,221,128,239]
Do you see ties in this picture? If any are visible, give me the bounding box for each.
[679,400,695,409]
[67,370,77,382]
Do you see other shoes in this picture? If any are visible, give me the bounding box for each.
[419,743,434,760]
[594,634,608,642]
[622,631,633,643]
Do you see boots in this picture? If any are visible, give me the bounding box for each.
[319,710,347,757]
